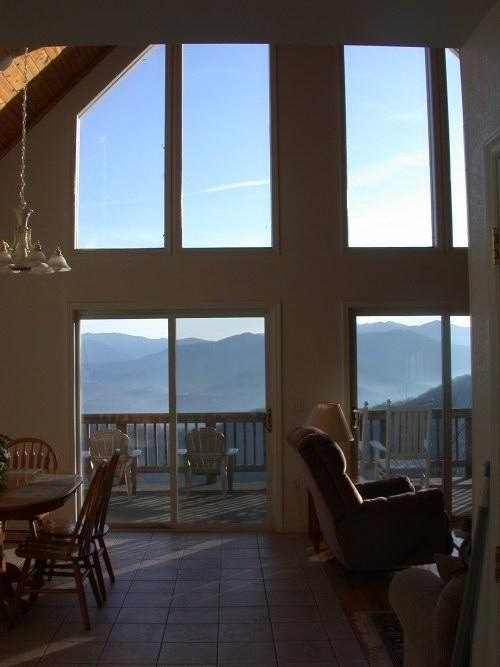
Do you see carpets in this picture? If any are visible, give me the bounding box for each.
[340,593,404,666]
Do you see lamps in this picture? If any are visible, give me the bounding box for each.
[304,401,355,443]
[0,48,72,276]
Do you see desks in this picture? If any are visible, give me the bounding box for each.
[308,490,320,553]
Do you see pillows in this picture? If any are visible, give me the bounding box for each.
[433,553,463,586]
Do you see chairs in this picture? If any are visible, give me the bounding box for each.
[0,437,121,630]
[390,562,466,667]
[88,431,141,500]
[370,400,433,488]
[287,425,453,615]
[184,427,238,494]
